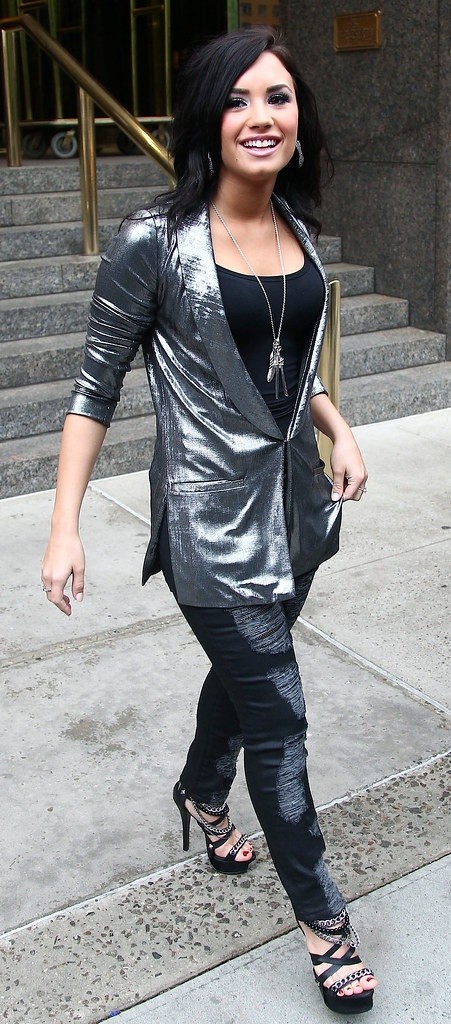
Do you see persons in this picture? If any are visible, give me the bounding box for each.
[41,28,377,1014]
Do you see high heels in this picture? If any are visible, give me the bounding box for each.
[172,780,256,875]
[297,905,377,1014]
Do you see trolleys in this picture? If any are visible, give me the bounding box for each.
[18,1,175,159]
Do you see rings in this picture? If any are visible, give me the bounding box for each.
[358,488,367,493]
[42,586,51,592]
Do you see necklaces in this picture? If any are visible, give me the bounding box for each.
[210,200,288,400]
